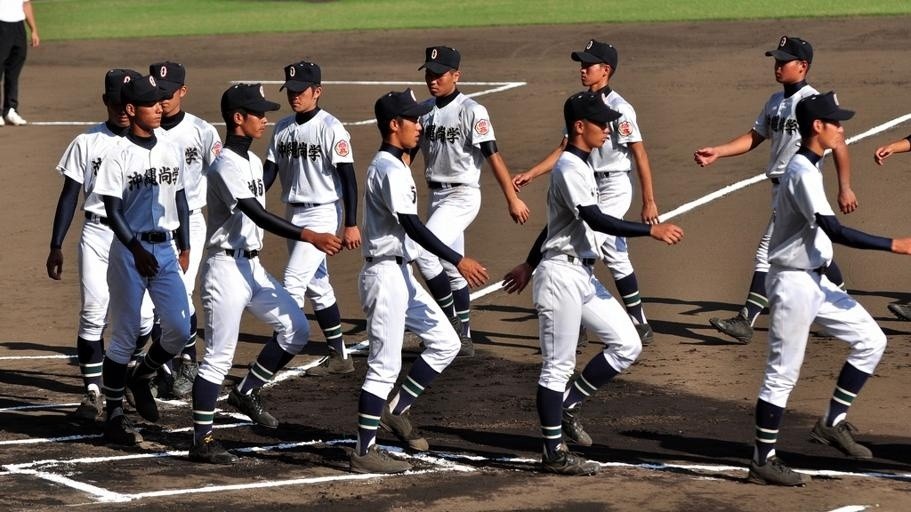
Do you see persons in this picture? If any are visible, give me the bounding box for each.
[0,1,39,127]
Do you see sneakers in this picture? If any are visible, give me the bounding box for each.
[101,406,144,446]
[0,106,28,127]
[302,350,357,378]
[186,432,240,466]
[346,444,414,476]
[807,415,875,462]
[885,301,910,321]
[558,404,598,449]
[417,316,476,359]
[65,389,104,429]
[745,455,814,489]
[708,310,755,347]
[537,450,602,480]
[161,357,200,399]
[376,403,431,453]
[121,366,161,423]
[632,322,655,349]
[226,383,281,431]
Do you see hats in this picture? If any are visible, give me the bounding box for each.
[568,38,620,72]
[793,89,856,125]
[219,82,281,114]
[560,90,623,125]
[99,60,186,107]
[277,60,322,94]
[372,85,434,120]
[762,34,814,64]
[417,45,462,76]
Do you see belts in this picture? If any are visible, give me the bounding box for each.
[364,254,403,266]
[563,255,598,267]
[81,209,112,228]
[793,264,826,278]
[768,174,785,186]
[591,169,632,180]
[130,230,177,245]
[425,179,462,190]
[285,201,323,210]
[222,247,260,261]
